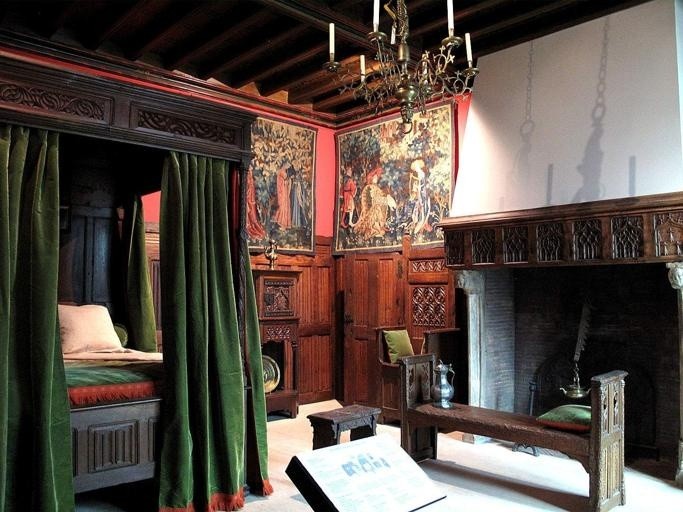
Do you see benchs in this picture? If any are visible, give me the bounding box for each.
[398,353,631,512]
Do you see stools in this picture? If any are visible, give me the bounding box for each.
[306,403,382,451]
[373,325,463,428]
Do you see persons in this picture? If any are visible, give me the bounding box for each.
[245,153,307,241]
[339,160,435,242]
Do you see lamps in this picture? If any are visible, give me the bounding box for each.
[320,0,483,133]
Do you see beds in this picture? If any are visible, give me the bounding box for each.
[1,298,268,512]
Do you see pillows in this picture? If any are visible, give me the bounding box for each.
[382,329,415,364]
[534,401,592,436]
[53,302,125,358]
